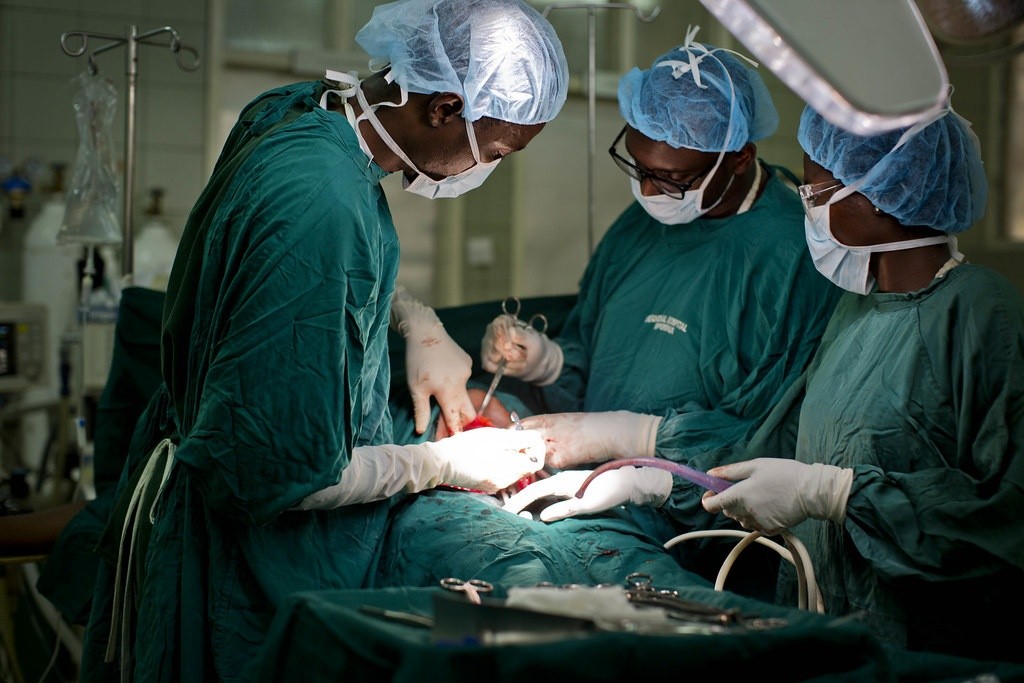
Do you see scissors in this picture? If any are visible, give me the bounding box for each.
[440,573,493,605]
[623,571,727,623]
[479,296,549,420]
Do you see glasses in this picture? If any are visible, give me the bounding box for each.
[798,178,842,222]
[609,121,727,200]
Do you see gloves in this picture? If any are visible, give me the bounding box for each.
[508,408,662,469]
[701,456,854,537]
[482,313,563,386]
[500,465,673,522]
[388,284,476,437]
[297,426,547,512]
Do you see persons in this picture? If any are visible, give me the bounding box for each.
[82,0,571,683]
[502,103,1024,662]
[481,40,843,597]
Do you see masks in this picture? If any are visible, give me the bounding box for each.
[391,119,503,198]
[628,153,735,225]
[805,184,950,295]
